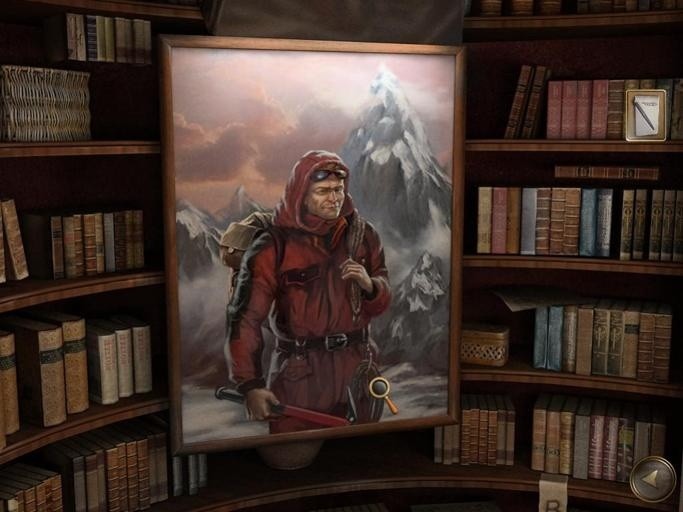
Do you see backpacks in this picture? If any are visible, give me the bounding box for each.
[219,211,273,299]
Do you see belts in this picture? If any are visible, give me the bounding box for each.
[278,329,368,352]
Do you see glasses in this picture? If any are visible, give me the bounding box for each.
[309,169,347,180]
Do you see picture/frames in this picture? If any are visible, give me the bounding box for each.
[155,35,469,458]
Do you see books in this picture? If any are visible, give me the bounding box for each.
[478,185,612,257]
[1,198,28,284]
[46,208,145,280]
[502,65,546,140]
[620,190,682,261]
[433,393,515,466]
[42,423,209,512]
[547,79,682,142]
[86,318,152,404]
[43,12,155,65]
[555,164,659,180]
[1,308,89,434]
[481,2,682,15]
[1,463,63,512]
[532,393,666,483]
[535,304,673,383]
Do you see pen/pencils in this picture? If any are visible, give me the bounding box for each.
[634,101,655,130]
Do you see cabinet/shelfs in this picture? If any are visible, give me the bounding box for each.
[1,0,683,511]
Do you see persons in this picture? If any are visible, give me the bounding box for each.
[224,149,392,433]
